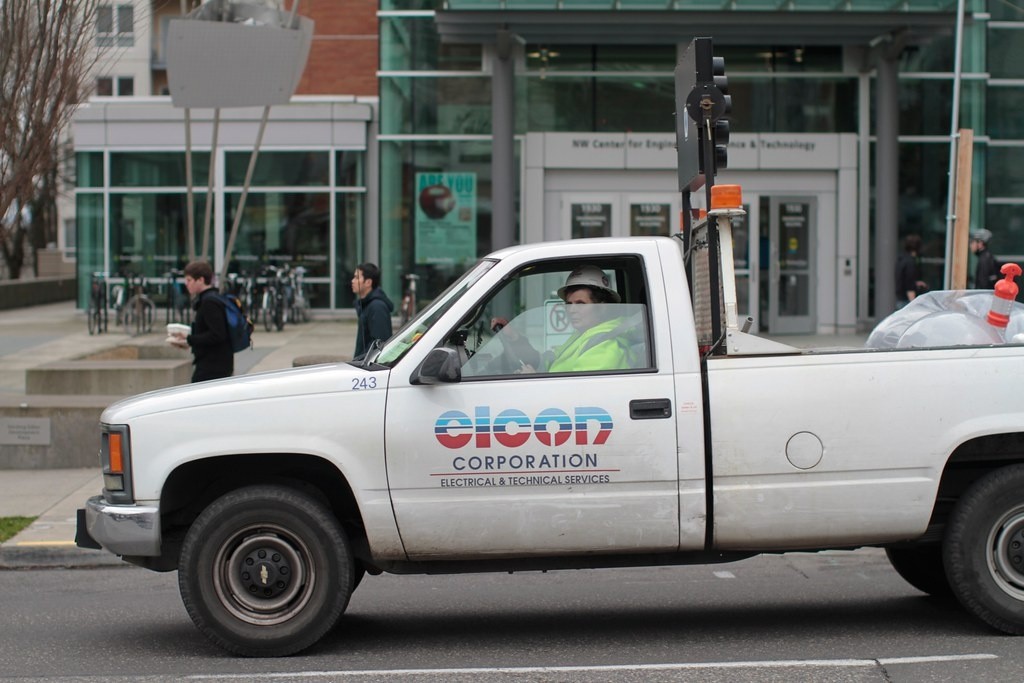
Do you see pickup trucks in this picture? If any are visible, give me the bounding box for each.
[73,186,1024,658]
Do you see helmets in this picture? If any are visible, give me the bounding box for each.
[557,267,621,302]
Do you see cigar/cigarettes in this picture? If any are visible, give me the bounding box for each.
[519,359,525,366]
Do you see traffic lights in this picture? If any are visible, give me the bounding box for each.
[696,57,728,173]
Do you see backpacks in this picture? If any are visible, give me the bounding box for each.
[201,293,254,354]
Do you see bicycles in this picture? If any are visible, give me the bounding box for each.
[399,274,419,323]
[84,262,307,335]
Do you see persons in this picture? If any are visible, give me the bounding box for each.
[489,266,641,374]
[351,262,394,359]
[895,234,926,310]
[170,260,234,383]
[969,228,1001,290]
